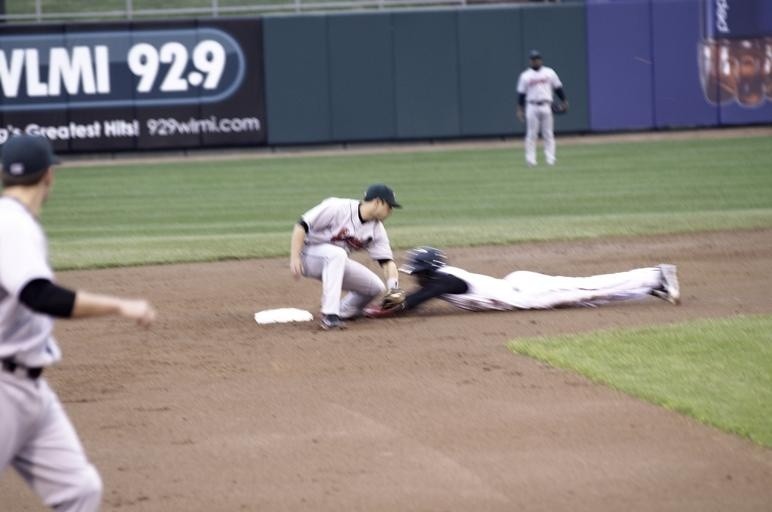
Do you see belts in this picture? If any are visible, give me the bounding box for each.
[0,357,42,379]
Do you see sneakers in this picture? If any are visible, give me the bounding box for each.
[319,311,345,330]
[648,264,680,304]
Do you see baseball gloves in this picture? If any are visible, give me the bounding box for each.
[381,291,404,317]
[552,102,568,115]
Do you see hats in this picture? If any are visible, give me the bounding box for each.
[528,49,541,58]
[365,185,401,207]
[0,135,61,177]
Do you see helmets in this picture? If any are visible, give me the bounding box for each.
[400,248,446,278]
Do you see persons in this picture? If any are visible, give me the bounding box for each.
[0,129,159,512]
[362,246,681,318]
[288,184,405,332]
[515,50,569,167]
